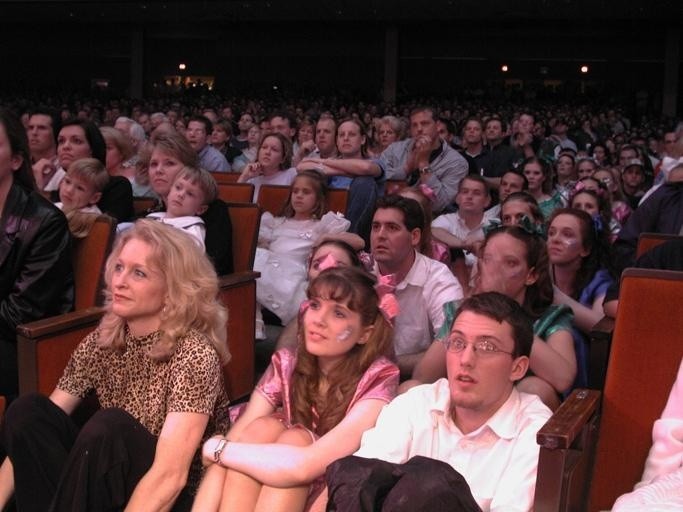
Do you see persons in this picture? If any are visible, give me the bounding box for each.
[209,117,242,165]
[167,110,185,131]
[575,175,602,194]
[620,159,645,210]
[483,117,513,175]
[221,105,240,135]
[535,118,578,163]
[592,168,631,227]
[253,168,365,341]
[26,110,62,167]
[185,115,231,173]
[150,113,167,128]
[31,117,132,220]
[619,146,639,167]
[366,193,464,379]
[114,116,145,156]
[655,129,676,175]
[520,156,556,219]
[0,100,72,405]
[500,111,539,174]
[571,190,625,273]
[430,175,495,266]
[619,121,683,242]
[303,113,340,161]
[296,118,386,198]
[546,205,612,332]
[306,239,360,282]
[611,359,683,512]
[190,266,401,512]
[116,166,218,255]
[232,112,300,176]
[500,192,544,233]
[308,292,554,512]
[379,105,469,211]
[552,154,576,209]
[242,123,262,161]
[53,157,110,217]
[98,126,135,183]
[576,159,598,180]
[134,128,235,276]
[366,115,403,159]
[235,112,256,150]
[399,224,577,411]
[603,237,683,318]
[462,117,489,176]
[0,218,232,512]
[236,132,298,203]
[136,112,156,137]
[437,117,465,156]
[482,170,528,231]
[588,142,609,166]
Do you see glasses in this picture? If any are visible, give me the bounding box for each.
[443,335,513,359]
[187,129,204,136]
[578,167,595,173]
[601,177,614,183]
[665,139,677,144]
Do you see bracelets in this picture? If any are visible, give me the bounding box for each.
[212,438,228,463]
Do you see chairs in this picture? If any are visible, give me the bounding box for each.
[19,214,118,396]
[257,185,349,220]
[135,196,157,216]
[216,182,255,204]
[589,233,683,391]
[210,171,241,181]
[215,202,263,404]
[529,268,683,512]
[386,178,407,195]
